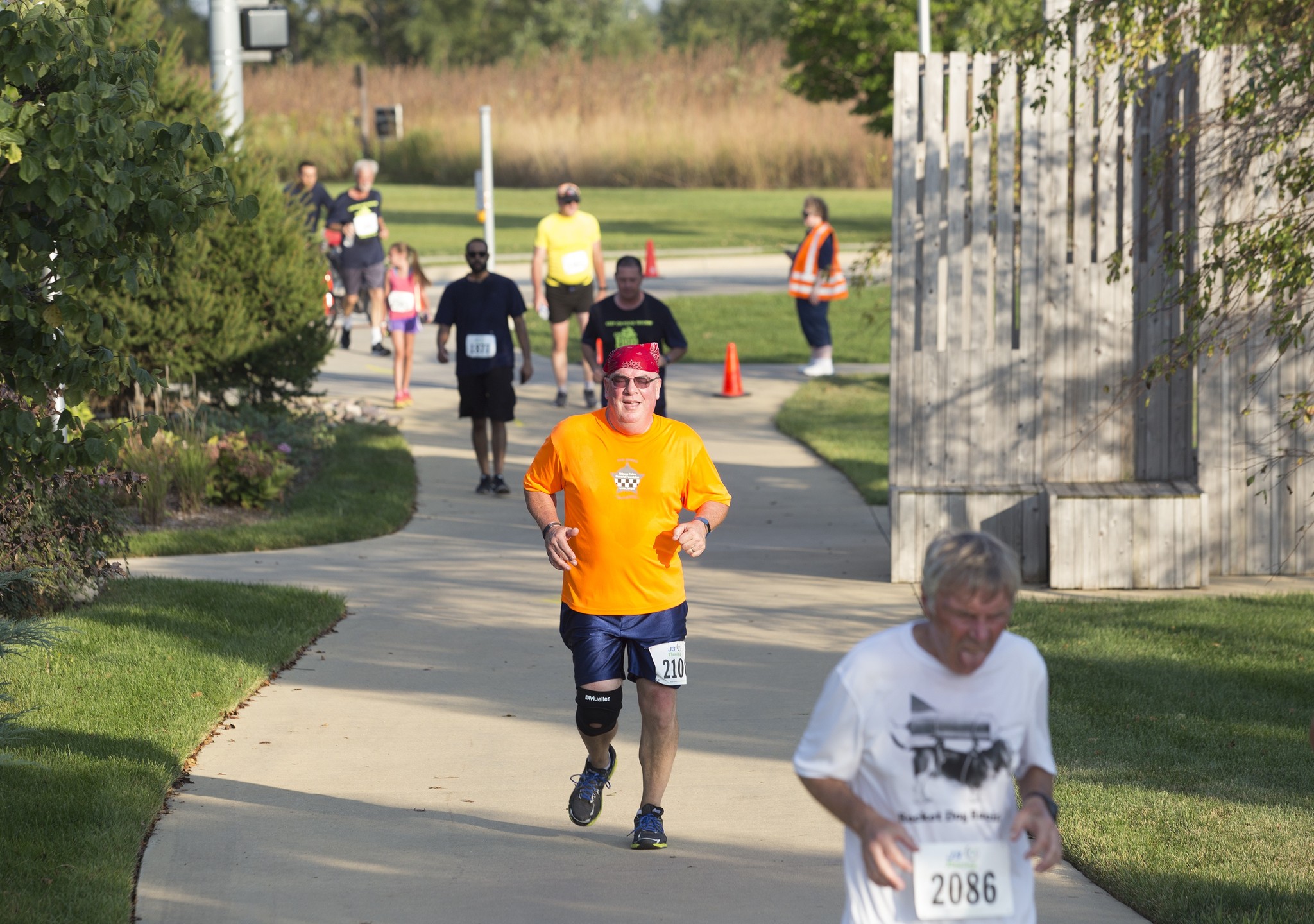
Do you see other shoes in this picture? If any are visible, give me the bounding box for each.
[584,389,598,408]
[394,396,403,408]
[373,343,389,355]
[341,325,350,348]
[555,391,567,408]
[801,358,833,377]
[403,392,412,404]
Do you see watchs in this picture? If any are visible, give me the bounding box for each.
[1022,790,1059,821]
[690,517,711,538]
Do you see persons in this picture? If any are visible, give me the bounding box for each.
[529,181,606,408]
[793,527,1063,924]
[433,238,532,495]
[523,342,732,850]
[780,196,851,376]
[579,255,689,419]
[279,157,432,411]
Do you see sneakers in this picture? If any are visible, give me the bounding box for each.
[626,803,667,849]
[568,743,616,827]
[477,472,493,495]
[493,475,509,493]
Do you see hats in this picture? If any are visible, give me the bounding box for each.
[557,182,581,203]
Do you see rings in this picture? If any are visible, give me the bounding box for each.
[691,548,695,553]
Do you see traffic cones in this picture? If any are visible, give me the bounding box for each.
[641,240,663,278]
[713,341,753,398]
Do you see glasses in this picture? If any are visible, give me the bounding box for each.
[803,212,816,217]
[609,376,660,389]
[469,251,487,256]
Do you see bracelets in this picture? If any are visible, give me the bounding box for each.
[663,353,671,364]
[599,287,609,290]
[543,522,562,540]
